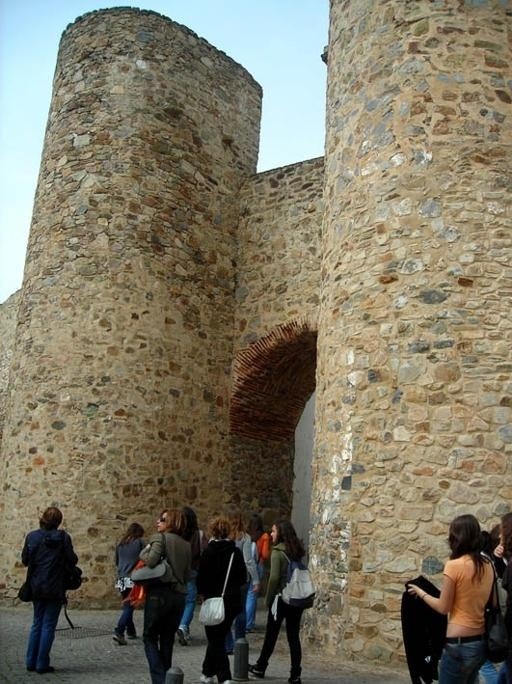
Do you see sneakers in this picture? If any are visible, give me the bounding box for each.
[127,634,139,639]
[177,627,193,646]
[199,674,214,684]
[223,679,232,684]
[112,632,128,645]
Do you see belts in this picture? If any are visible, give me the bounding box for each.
[443,634,482,659]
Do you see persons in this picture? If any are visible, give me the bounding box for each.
[113,506,303,684]
[407,514,510,684]
[21,507,74,674]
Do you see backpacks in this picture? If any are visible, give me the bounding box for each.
[274,544,315,620]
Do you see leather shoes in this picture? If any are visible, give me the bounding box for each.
[288,677,302,684]
[27,663,36,671]
[247,663,265,679]
[36,665,54,673]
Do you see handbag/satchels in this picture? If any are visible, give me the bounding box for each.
[60,529,83,590]
[15,565,34,602]
[481,551,512,663]
[199,597,226,627]
[130,531,178,585]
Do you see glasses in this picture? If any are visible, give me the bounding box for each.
[159,517,166,522]
